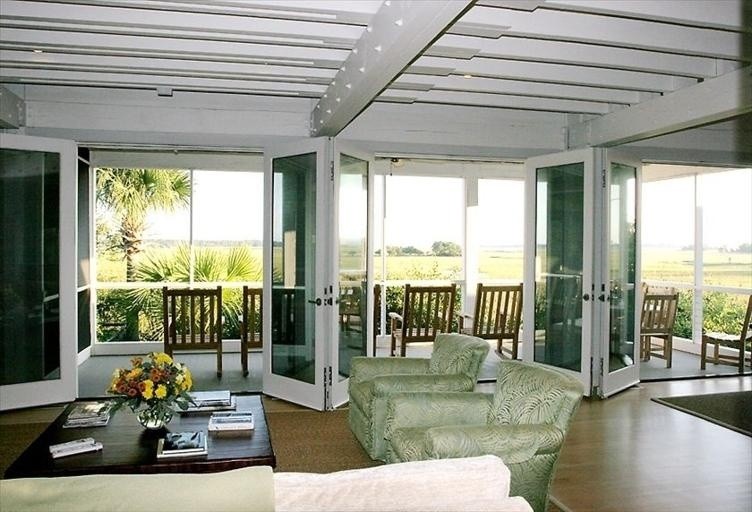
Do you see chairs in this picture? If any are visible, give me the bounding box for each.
[386,358,585,512]
[242,285,295,379]
[641,281,676,298]
[389,282,458,353]
[462,284,524,363]
[160,285,223,378]
[639,292,679,369]
[348,333,489,457]
[702,293,750,378]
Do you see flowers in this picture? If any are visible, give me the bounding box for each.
[106,351,198,416]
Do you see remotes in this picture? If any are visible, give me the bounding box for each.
[49,437,94,453]
[52,442,103,459]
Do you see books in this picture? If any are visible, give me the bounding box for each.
[174,390,254,432]
[155,438,208,458]
[62,404,111,429]
[161,432,205,452]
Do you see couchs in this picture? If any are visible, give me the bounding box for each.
[0,455,533,512]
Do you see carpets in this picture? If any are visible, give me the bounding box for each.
[650,390,752,440]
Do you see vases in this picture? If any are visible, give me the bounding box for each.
[135,401,170,430]
